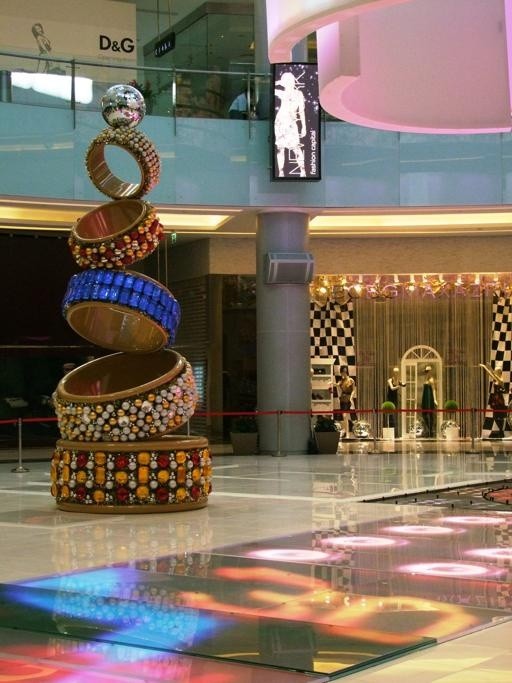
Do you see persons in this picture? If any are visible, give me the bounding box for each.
[382,367,406,437]
[327,365,358,432]
[228,86,254,119]
[421,366,438,437]
[274,72,307,177]
[479,363,506,431]
[31,22,66,75]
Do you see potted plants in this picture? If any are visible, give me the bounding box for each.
[228,416,261,455]
[379,401,396,438]
[312,415,344,454]
[442,399,461,440]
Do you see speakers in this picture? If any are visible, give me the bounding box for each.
[264,252,314,285]
